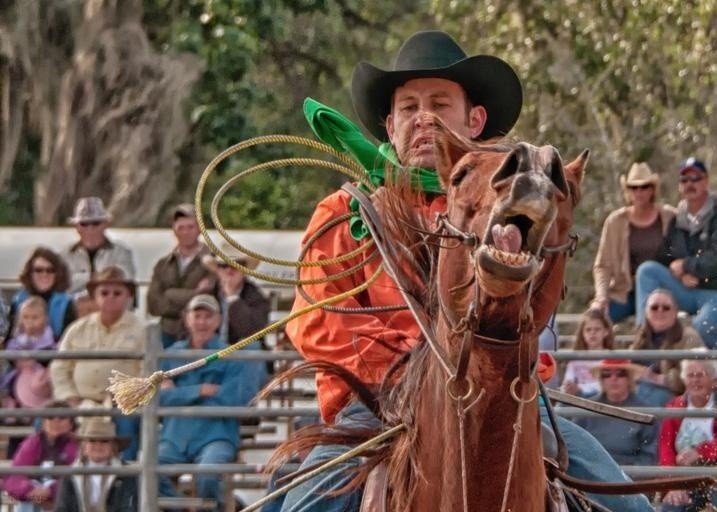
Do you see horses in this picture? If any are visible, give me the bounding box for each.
[246,112,590,512]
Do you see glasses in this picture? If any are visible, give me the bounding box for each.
[628,185,649,189]
[649,303,672,311]
[32,267,56,274]
[601,368,627,378]
[678,175,705,183]
[99,289,124,297]
[80,221,102,226]
[215,261,248,269]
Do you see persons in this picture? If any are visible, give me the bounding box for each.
[53,197,134,298]
[658,347,717,512]
[634,156,716,348]
[553,309,615,408]
[146,202,217,349]
[0,241,276,512]
[280,29,657,512]
[566,357,659,468]
[585,161,676,327]
[627,289,706,408]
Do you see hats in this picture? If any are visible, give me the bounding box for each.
[16,368,55,408]
[351,30,523,143]
[74,417,130,450]
[203,235,260,273]
[679,157,707,174]
[86,265,138,295]
[173,204,195,218]
[590,359,643,380]
[619,162,661,195]
[188,294,220,313]
[67,197,112,224]
